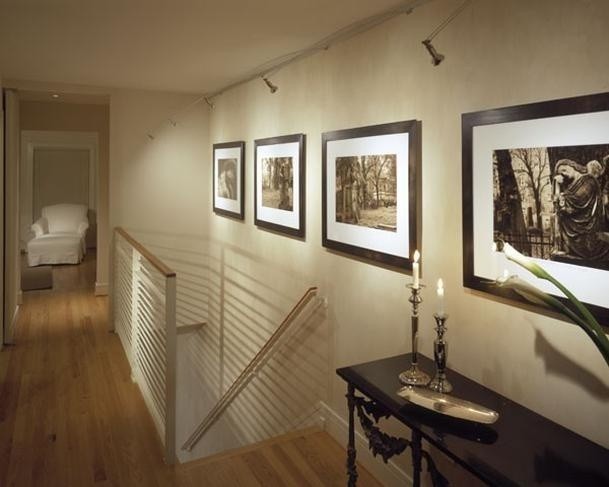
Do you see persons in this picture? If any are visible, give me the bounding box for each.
[551,157,608,259]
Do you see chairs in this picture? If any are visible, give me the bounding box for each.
[26,202,91,267]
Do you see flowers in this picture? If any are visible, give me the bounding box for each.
[480,237,608,365]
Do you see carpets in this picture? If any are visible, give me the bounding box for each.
[21,252,53,290]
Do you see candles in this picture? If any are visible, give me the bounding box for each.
[436,277,444,316]
[412,249,419,286]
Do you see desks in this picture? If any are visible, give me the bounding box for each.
[336,351,608,486]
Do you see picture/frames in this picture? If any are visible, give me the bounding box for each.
[322,119,417,270]
[462,92,609,327]
[212,131,307,238]
